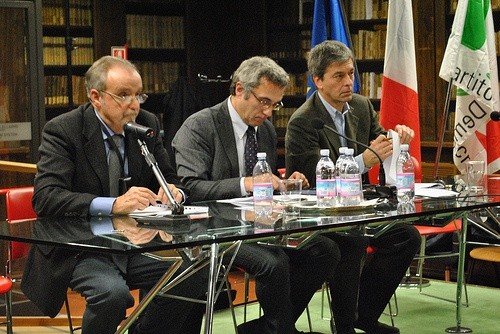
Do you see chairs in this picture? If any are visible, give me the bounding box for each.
[0,168,500,334]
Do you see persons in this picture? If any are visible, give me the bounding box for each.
[194,201,282,225]
[171,57,342,334]
[284,40,421,334]
[21,56,204,334]
[37,216,173,244]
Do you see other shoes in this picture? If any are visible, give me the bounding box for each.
[356,320,400,334]
[330,319,356,334]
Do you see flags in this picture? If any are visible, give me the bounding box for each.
[306,0,360,100]
[439,0,500,181]
[378,0,423,182]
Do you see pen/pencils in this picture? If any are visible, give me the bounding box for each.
[207,225,251,232]
[370,137,393,144]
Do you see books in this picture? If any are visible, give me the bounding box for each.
[271,1,385,135]
[41,0,185,104]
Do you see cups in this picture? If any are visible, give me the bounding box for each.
[282,216,301,231]
[277,179,304,217]
[465,161,485,193]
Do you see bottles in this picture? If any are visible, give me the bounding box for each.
[340,149,362,206]
[316,148,336,208]
[253,217,274,234]
[253,152,274,217]
[395,144,415,204]
[397,203,416,215]
[336,147,349,206]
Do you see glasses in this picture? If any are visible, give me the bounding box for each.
[249,89,283,110]
[101,90,148,104]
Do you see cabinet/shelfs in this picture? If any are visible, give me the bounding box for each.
[260,0,447,180]
[0,0,191,223]
[0,0,46,277]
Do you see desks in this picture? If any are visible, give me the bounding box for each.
[0,176,500,334]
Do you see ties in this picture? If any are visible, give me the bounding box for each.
[245,126,258,177]
[109,136,128,273]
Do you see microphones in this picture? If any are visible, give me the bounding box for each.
[122,124,154,138]
[312,118,397,200]
